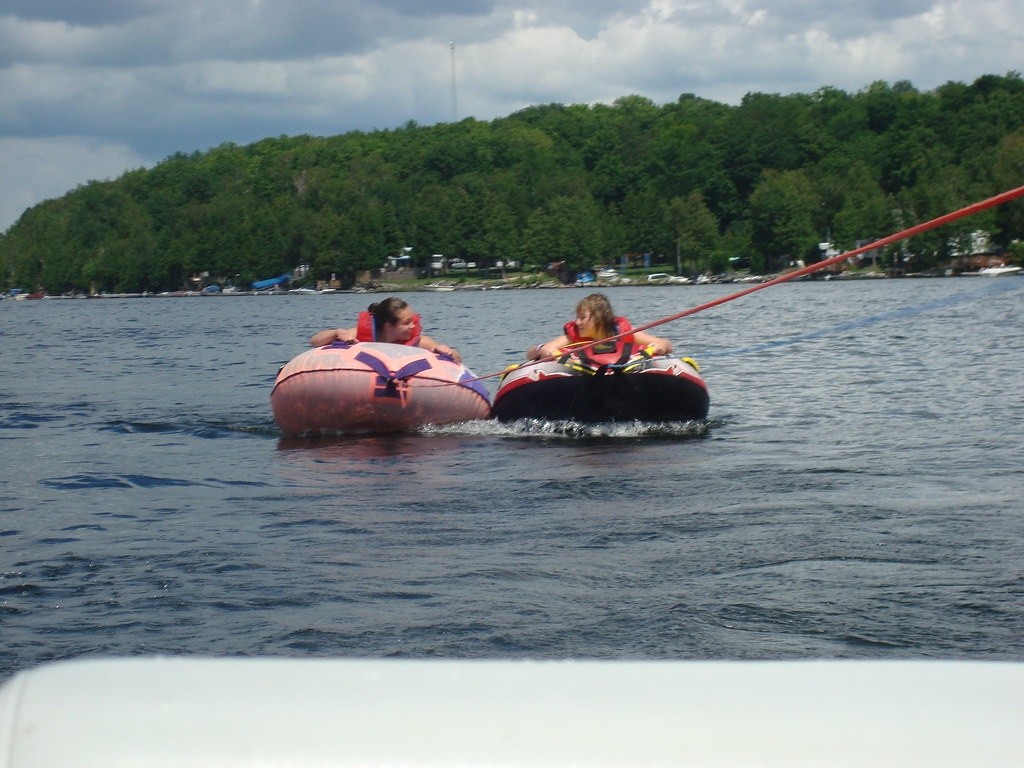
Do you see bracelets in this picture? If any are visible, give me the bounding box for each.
[536,344,544,351]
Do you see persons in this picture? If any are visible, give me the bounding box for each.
[526,294,673,371]
[310,297,462,363]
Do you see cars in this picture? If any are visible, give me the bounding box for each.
[647,273,683,281]
[431,253,521,269]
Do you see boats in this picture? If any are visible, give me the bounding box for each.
[268,339,492,435]
[492,344,711,421]
